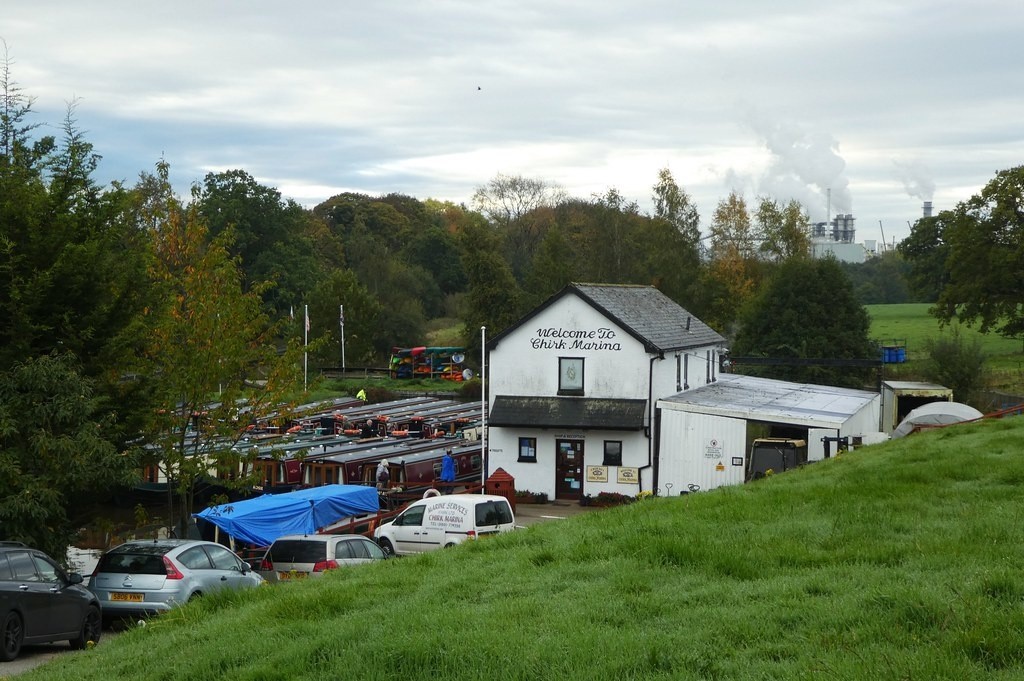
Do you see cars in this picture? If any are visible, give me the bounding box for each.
[87,539,267,628]
[0,541,103,661]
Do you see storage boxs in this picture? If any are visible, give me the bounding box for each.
[883,345,906,363]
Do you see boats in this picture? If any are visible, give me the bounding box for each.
[127,395,489,504]
[120,479,192,504]
[260,533,390,583]
[192,482,408,569]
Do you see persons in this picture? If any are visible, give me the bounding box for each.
[356,388,369,404]
[342,415,351,430]
[375,459,389,489]
[440,449,456,495]
[360,420,372,439]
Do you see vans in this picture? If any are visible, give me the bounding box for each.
[374,493,515,558]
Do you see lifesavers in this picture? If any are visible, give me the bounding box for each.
[239,424,255,433]
[286,425,302,433]
[334,412,343,422]
[410,416,424,421]
[430,431,445,438]
[344,430,361,435]
[422,488,442,499]
[453,372,464,383]
[391,430,408,437]
[377,415,391,423]
